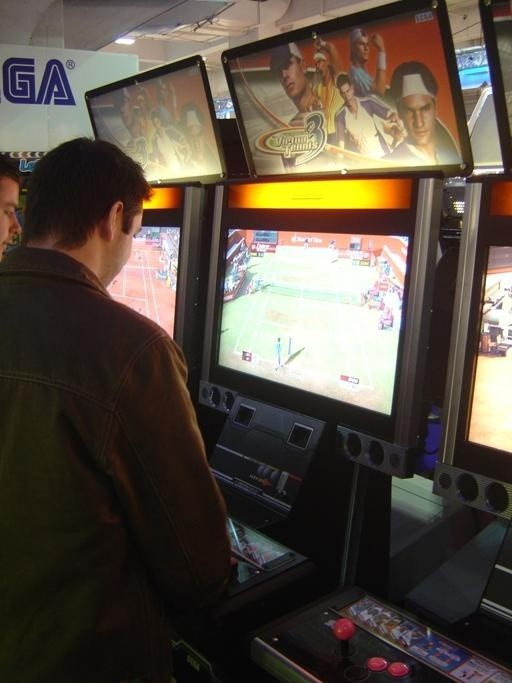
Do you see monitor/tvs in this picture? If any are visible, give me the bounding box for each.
[216,224,410,432]
[104,213,179,358]
[462,245,512,469]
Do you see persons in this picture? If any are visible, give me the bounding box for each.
[0,150,22,263]
[1,135,269,683]
[119,79,206,180]
[269,24,462,173]
